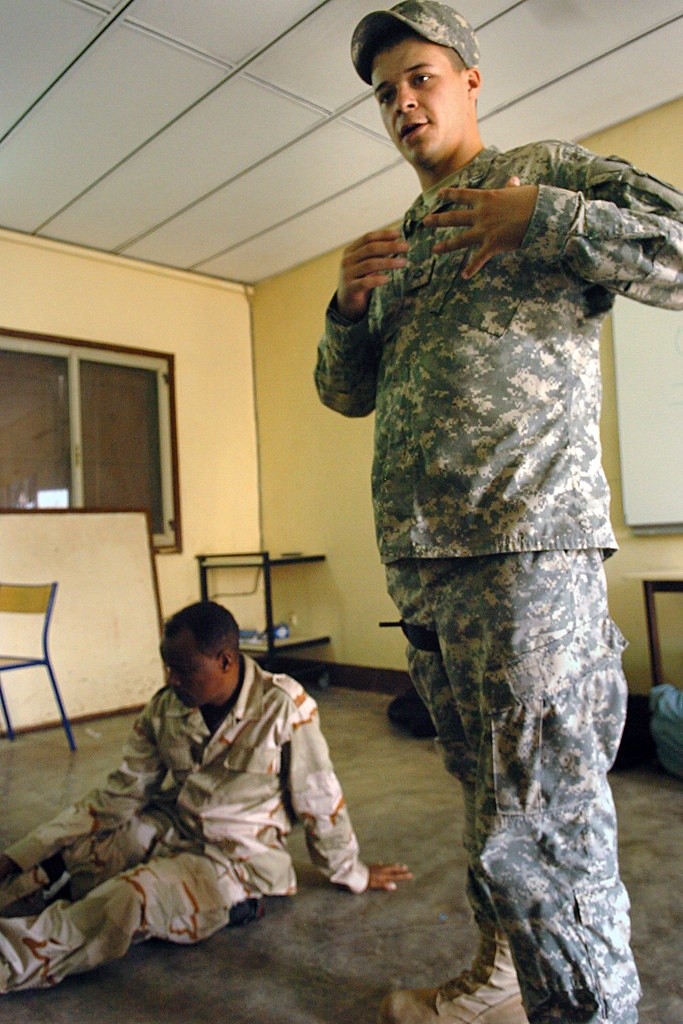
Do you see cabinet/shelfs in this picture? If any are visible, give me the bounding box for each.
[195,552,331,681]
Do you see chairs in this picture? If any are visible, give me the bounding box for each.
[0,581,76,750]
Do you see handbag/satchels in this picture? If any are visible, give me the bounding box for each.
[651,681,683,777]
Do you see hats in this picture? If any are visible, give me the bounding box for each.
[350,0,481,85]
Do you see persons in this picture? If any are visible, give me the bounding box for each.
[0,601,410,994]
[318,0,683,1024]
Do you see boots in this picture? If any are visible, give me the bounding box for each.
[377,926,532,1024]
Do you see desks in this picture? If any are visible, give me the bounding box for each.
[623,570,683,691]
